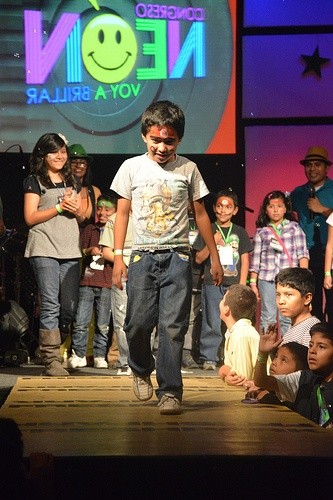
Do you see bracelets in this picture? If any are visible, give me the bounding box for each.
[256,353,267,363]
[324,272,331,276]
[114,249,123,256]
[56,205,62,213]
[83,249,87,257]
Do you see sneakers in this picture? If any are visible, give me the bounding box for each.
[62,349,87,368]
[132,373,153,401]
[94,357,108,368]
[203,360,216,370]
[159,392,182,416]
[182,352,198,368]
[117,367,131,375]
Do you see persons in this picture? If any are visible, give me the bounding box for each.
[244,268,333,431]
[22,133,86,376]
[0,144,205,376]
[288,146,333,325]
[219,284,260,386]
[110,101,224,414]
[249,191,310,336]
[192,189,254,370]
[0,418,54,500]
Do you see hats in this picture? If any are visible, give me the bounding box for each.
[299,147,332,166]
[67,144,90,158]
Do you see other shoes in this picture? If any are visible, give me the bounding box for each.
[109,360,122,369]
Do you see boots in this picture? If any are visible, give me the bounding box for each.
[35,332,73,361]
[38,328,70,376]
